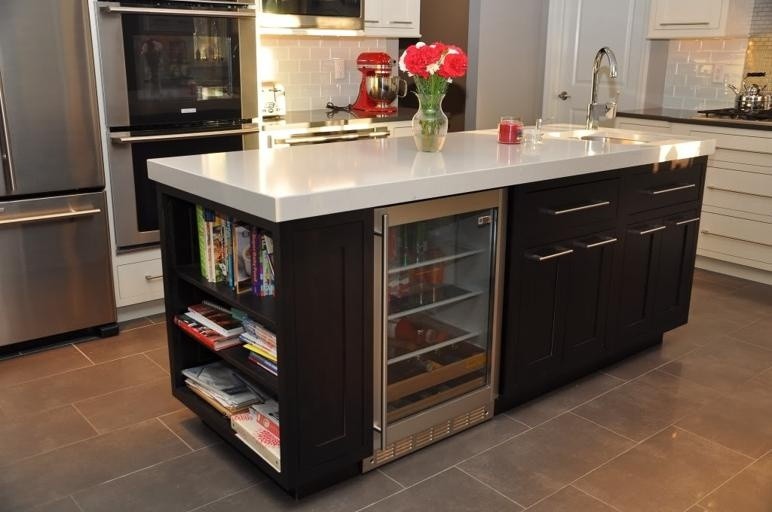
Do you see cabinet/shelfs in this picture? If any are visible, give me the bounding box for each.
[540,1,667,131]
[364,0,421,39]
[500,176,618,405]
[619,154,710,366]
[156,184,374,488]
[651,1,755,39]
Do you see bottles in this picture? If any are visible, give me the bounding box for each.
[498,117,524,144]
[388,227,448,373]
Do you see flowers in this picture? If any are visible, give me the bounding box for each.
[398,39,468,134]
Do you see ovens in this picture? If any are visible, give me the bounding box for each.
[89,2,259,249]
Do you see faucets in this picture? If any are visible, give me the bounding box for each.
[585,46,619,131]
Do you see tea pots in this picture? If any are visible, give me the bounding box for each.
[728,69,772,117]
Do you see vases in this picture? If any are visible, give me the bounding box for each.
[411,93,448,152]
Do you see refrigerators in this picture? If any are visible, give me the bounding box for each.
[1,0,117,352]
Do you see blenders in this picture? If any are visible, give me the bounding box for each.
[354,52,407,115]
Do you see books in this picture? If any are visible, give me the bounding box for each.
[173,203,282,474]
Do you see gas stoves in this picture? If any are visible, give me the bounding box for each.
[698,99,771,123]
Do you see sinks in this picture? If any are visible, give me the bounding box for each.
[581,135,652,146]
